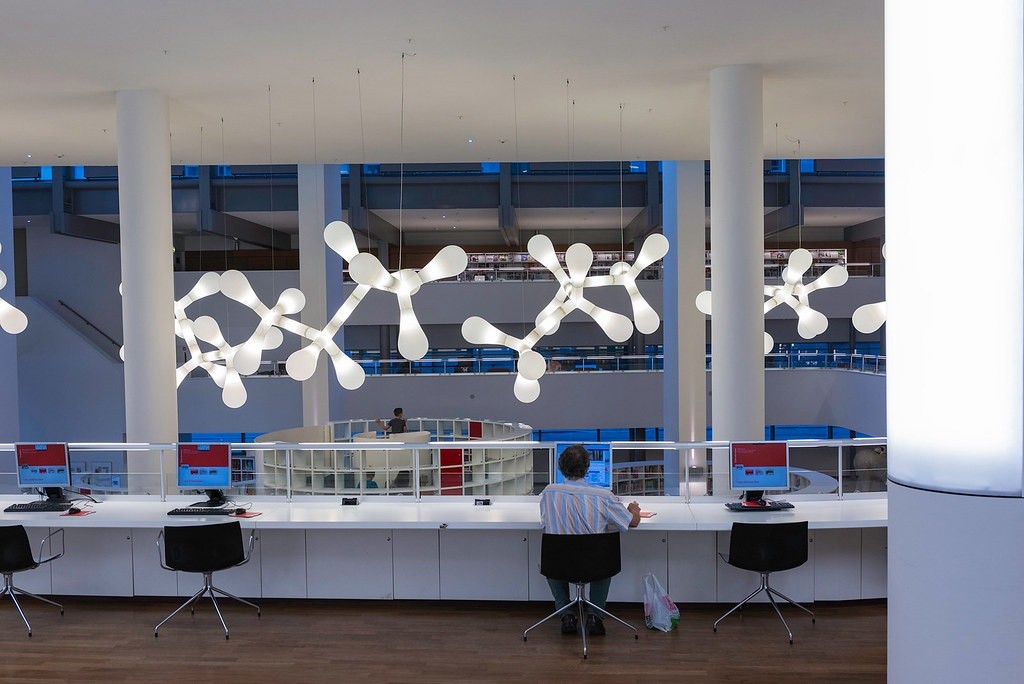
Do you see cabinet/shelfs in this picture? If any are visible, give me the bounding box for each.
[455,248,848,282]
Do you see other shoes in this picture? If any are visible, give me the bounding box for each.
[585,614,606,636]
[561,614,578,634]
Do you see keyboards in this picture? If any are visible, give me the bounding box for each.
[725,501,796,511]
[4,504,74,513]
[167,508,235,515]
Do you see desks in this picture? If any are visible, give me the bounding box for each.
[0,492,888,620]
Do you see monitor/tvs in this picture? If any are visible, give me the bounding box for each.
[729,440,790,500]
[176,442,232,506]
[13,441,72,504]
[554,440,613,491]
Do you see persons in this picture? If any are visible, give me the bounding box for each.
[374,407,408,433]
[388,471,413,495]
[538,447,641,633]
[357,471,379,495]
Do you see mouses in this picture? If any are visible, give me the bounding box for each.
[69,508,81,515]
[757,499,766,506]
[236,508,247,514]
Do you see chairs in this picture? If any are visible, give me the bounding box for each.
[523,532,639,659]
[152,520,261,641]
[0,525,68,637]
[712,521,808,647]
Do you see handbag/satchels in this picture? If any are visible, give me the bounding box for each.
[642,573,680,632]
